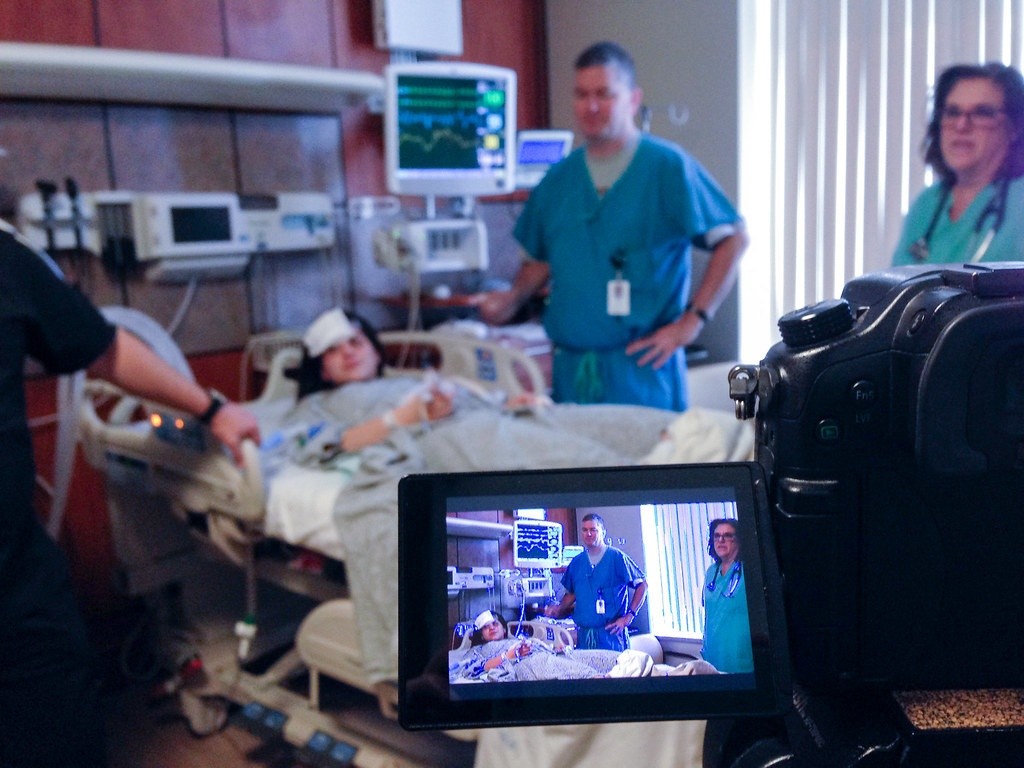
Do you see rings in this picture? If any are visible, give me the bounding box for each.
[614,626,619,630]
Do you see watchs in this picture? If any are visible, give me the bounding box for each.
[683,302,711,325]
[198,387,226,428]
[626,609,637,618]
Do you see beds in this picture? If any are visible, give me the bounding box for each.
[448,620,730,685]
[73,327,760,767]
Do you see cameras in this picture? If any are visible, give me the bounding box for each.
[398,261,1023,732]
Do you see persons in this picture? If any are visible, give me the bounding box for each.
[0,220,265,767]
[473,40,750,413]
[543,513,649,653]
[699,518,755,673]
[270,305,545,466]
[886,62,1024,267]
[463,608,610,679]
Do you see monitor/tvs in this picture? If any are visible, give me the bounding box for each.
[385,62,516,193]
[514,131,576,191]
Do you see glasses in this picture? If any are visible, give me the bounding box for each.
[712,532,736,543]
[942,106,1007,128]
